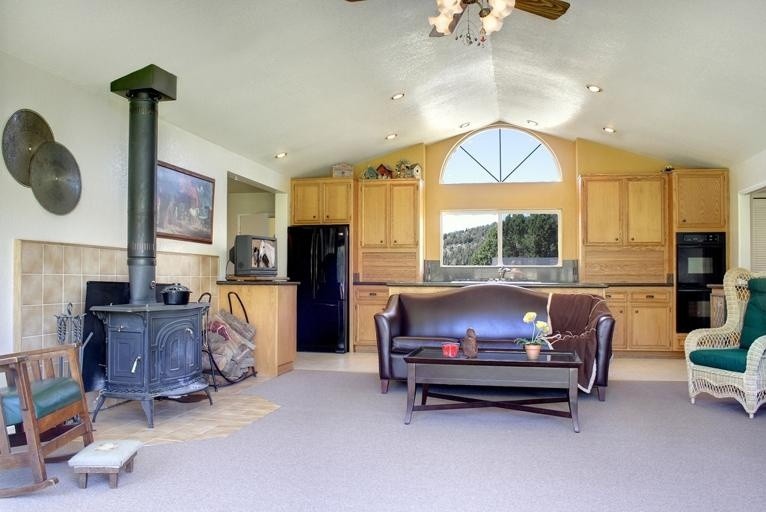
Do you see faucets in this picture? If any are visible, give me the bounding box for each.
[499,268,511,282]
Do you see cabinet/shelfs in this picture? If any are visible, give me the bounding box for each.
[216,279,301,376]
[346,284,387,354]
[352,178,425,283]
[290,176,355,226]
[596,289,673,353]
[577,172,669,285]
[672,167,728,232]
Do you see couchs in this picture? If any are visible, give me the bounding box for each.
[375,284,615,401]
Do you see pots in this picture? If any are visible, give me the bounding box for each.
[159,282,191,305]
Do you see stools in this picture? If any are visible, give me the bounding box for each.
[68,440,138,489]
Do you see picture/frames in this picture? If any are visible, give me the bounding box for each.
[153,157,213,244]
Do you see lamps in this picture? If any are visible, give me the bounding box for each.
[428,0,517,48]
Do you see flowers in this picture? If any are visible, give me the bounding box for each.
[513,309,549,345]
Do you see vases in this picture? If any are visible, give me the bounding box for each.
[524,344,541,360]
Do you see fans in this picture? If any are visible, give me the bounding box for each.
[345,0,571,35]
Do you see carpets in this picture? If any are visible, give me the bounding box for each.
[0,369,766,512]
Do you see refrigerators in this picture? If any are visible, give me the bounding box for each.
[287,224,351,353]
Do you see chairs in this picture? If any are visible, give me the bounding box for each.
[0,343,91,503]
[683,265,766,420]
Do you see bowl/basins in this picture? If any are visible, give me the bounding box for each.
[441,341,460,359]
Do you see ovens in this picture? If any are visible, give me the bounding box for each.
[677,232,726,332]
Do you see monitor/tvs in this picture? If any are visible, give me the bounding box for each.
[229,235,278,276]
